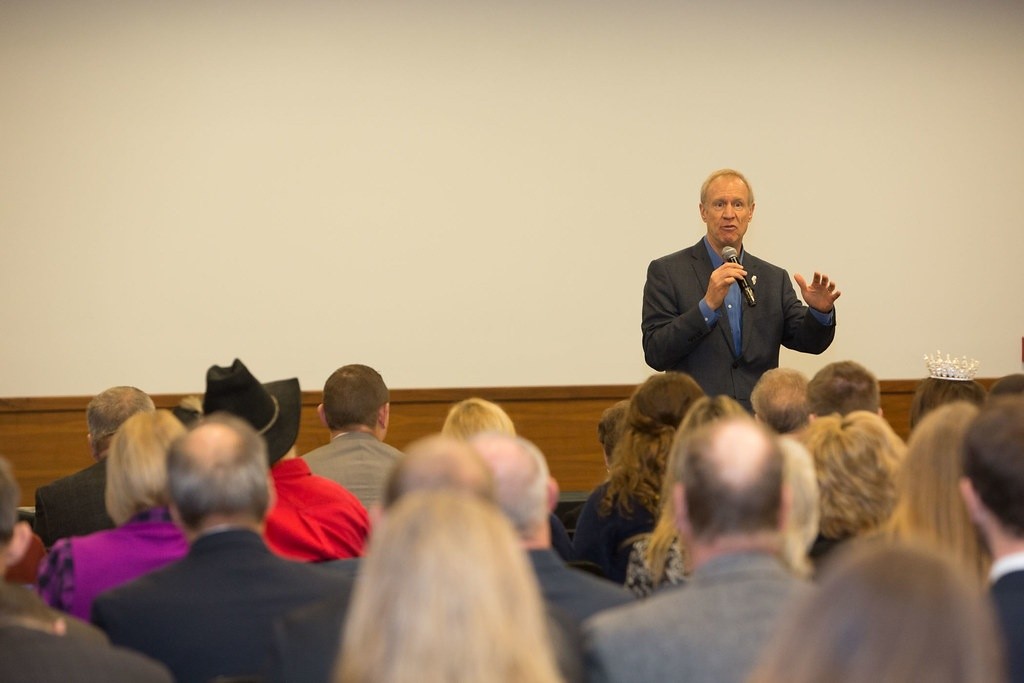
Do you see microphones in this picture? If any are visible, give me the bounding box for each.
[722,246,757,307]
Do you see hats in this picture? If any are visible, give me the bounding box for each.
[200,358,302,465]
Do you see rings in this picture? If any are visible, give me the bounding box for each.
[827,289,830,293]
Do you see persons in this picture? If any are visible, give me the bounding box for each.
[0,352,1024,683]
[641,168,842,417]
